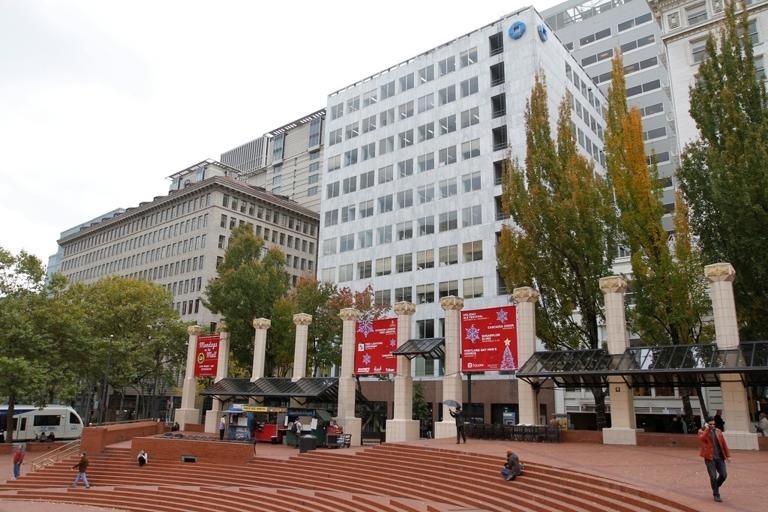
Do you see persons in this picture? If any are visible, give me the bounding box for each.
[39,431,47,442]
[753,412,768,437]
[500,450,521,481]
[448,406,467,444]
[292,417,302,449]
[174,421,180,430]
[714,409,726,433]
[219,414,227,440]
[248,414,258,437]
[696,415,732,504]
[670,416,684,433]
[71,452,90,488]
[47,431,54,441]
[136,448,148,467]
[12,443,24,480]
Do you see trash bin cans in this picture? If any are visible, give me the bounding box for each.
[299,435,318,453]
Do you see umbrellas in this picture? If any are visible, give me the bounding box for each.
[443,399,462,411]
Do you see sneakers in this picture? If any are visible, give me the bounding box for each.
[715,496,722,503]
